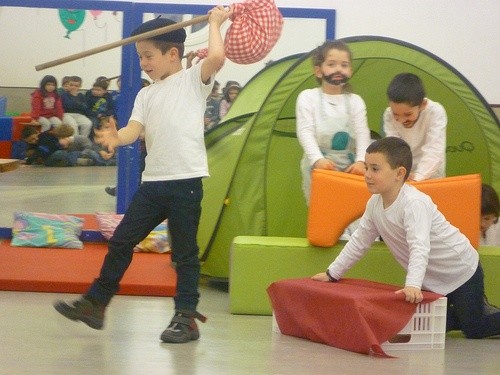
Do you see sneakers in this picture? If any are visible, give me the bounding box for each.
[161,307,208,344]
[53,297,106,330]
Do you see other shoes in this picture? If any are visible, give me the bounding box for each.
[104,185,118,195]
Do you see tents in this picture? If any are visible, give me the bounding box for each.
[168,36,500,283]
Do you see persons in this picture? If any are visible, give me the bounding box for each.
[21,75,150,167]
[383,73,448,182]
[52,3,236,343]
[205,81,220,132]
[313,136,500,339]
[477,184,500,248]
[220,81,242,122]
[295,41,372,240]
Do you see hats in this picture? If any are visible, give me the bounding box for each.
[224,81,242,103]
[132,14,186,44]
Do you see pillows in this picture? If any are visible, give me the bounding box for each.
[10,210,86,249]
[96,211,172,253]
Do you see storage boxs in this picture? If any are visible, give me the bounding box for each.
[272,278,447,351]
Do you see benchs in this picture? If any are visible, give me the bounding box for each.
[308,167,482,248]
[229,236,500,336]
